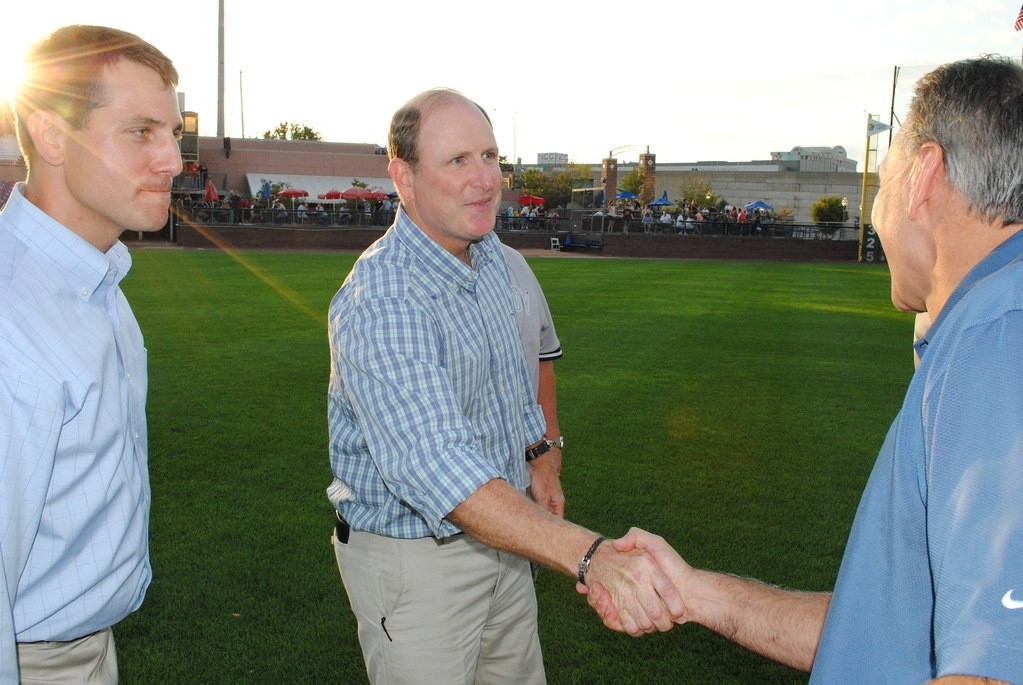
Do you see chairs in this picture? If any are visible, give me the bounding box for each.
[551,238,561,252]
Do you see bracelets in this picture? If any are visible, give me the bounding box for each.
[579,538,605,584]
[525,442,549,462]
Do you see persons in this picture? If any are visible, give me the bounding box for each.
[0,25,185,685]
[496,242,566,472]
[572,55,1023,685]
[662,198,768,233]
[178,160,399,226]
[507,201,560,231]
[620,201,635,234]
[606,199,620,231]
[640,204,655,232]
[326,89,688,685]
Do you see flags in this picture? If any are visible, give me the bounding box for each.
[1015,5,1023,32]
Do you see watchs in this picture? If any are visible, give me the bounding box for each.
[546,433,564,450]
